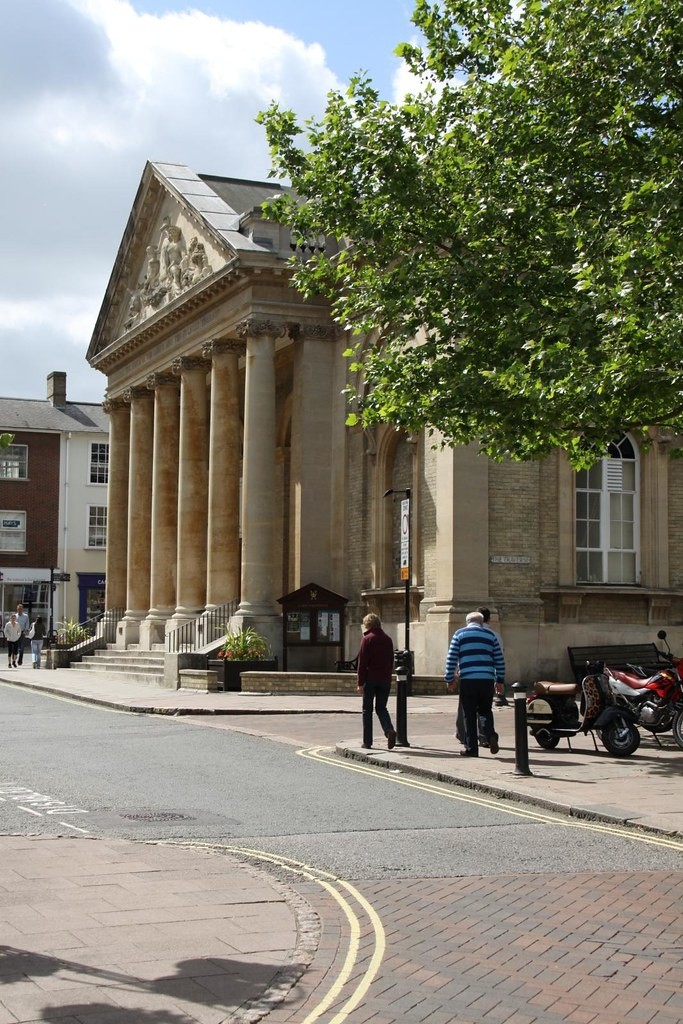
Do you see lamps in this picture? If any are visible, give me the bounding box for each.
[383,486,411,499]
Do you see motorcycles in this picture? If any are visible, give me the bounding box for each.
[601,630,683,749]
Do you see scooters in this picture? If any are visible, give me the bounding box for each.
[527,658,641,757]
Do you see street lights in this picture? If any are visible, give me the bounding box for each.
[384,486,411,745]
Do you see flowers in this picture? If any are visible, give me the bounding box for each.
[213,624,275,661]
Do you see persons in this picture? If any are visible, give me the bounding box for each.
[455,607,503,748]
[15,604,29,665]
[444,612,505,757]
[4,615,21,668]
[25,616,46,669]
[144,226,185,283]
[356,614,397,750]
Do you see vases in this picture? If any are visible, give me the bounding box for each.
[222,659,273,691]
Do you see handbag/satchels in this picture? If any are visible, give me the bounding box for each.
[28,623,35,639]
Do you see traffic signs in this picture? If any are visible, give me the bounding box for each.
[33,572,70,591]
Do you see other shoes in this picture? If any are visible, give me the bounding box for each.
[480,742,489,747]
[18,661,22,665]
[13,663,16,667]
[361,744,370,749]
[461,749,478,757]
[388,730,396,749]
[9,664,11,668]
[33,662,37,669]
[490,735,499,754]
[456,733,465,744]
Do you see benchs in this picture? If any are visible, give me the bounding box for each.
[568,644,670,687]
